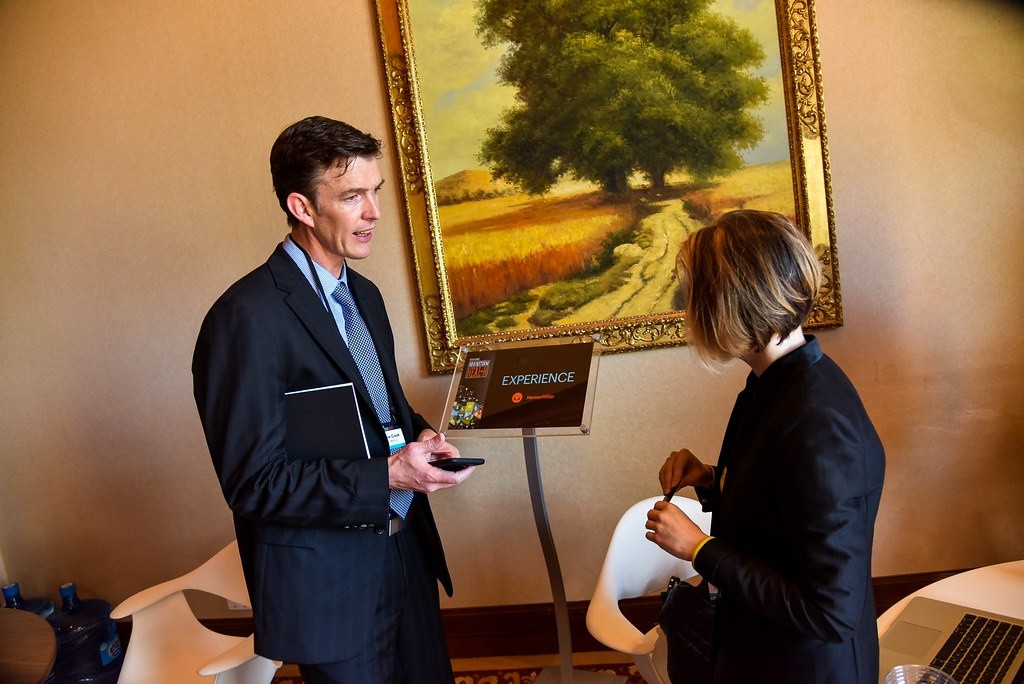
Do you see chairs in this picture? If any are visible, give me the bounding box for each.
[110,538,284,684]
[586,494,710,684]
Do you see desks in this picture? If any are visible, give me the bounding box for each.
[0,606,58,684]
[876,559,1024,642]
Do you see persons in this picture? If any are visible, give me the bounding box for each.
[646,210,886,684]
[191,116,478,684]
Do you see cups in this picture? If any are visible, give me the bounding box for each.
[886,665,958,684]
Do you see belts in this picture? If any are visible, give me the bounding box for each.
[387,513,409,536]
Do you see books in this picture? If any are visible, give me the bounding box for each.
[285,382,371,460]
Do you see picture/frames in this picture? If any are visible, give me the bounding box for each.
[374,0,841,376]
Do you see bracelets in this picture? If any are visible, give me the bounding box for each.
[691,536,715,568]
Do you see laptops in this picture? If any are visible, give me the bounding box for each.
[877,596,1024,684]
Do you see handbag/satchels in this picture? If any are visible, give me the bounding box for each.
[656,577,716,684]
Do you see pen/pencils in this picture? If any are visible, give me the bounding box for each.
[662,480,682,502]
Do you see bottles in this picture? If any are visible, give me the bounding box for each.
[2,583,58,684]
[48,583,124,684]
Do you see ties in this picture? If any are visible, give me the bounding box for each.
[331,282,414,519]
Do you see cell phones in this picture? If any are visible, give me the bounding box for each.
[427,458,485,468]
